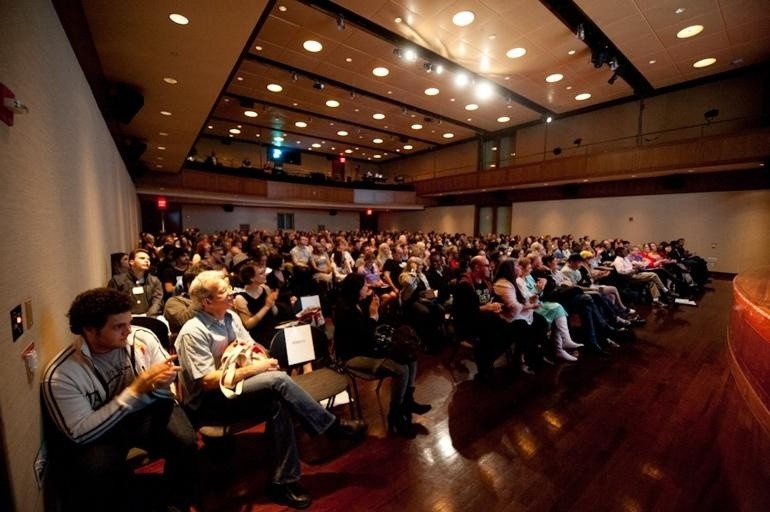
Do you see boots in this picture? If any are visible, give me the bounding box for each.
[387,402,415,440]
[403,386,431,419]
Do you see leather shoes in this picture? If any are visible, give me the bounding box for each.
[340,420,367,431]
[286,492,311,507]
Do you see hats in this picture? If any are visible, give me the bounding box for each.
[233,254,248,266]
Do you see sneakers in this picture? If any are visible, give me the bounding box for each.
[521,290,679,375]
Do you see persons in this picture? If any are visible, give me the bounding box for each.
[107,228,716,512]
[40,287,205,511]
[205,150,220,166]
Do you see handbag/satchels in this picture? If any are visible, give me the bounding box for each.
[375,323,420,365]
[220,339,267,399]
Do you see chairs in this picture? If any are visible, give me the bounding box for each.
[329,331,398,428]
[262,324,365,462]
[131,314,171,354]
